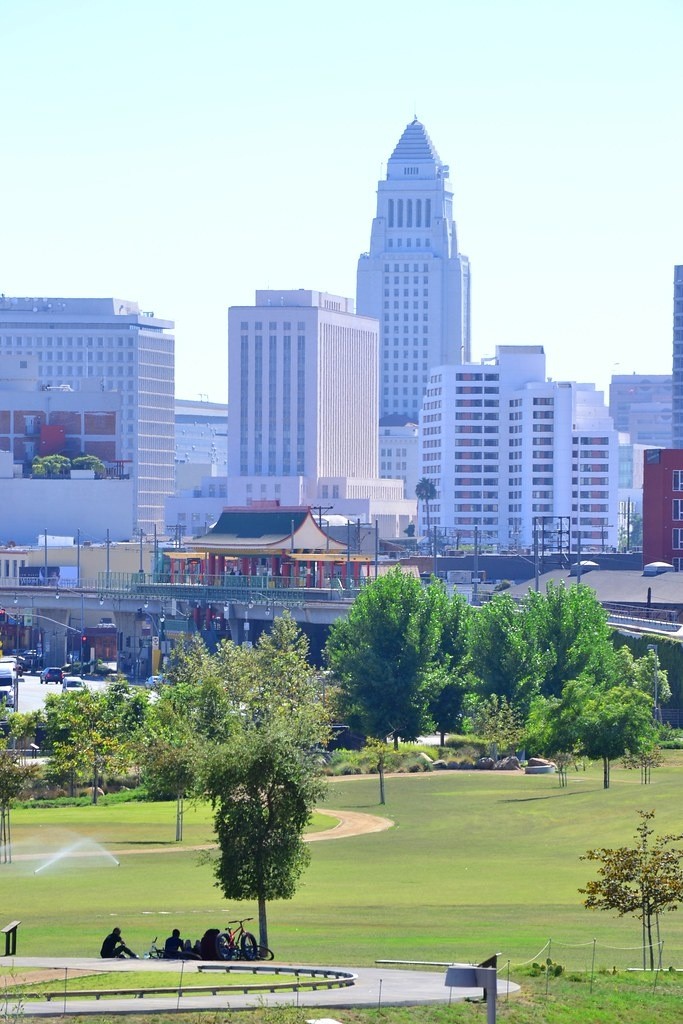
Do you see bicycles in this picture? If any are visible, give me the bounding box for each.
[148,937,202,960]
[214,918,274,961]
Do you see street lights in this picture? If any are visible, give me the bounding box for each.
[99,592,120,674]
[142,595,191,672]
[221,591,305,650]
[647,644,658,720]
[13,591,36,650]
[55,586,84,678]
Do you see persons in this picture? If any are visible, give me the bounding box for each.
[163,929,202,960]
[230,568,243,587]
[100,928,139,958]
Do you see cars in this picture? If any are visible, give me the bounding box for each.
[61,676,86,697]
[39,667,64,684]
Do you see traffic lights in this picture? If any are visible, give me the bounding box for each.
[82,635,88,642]
[0,608,5,621]
